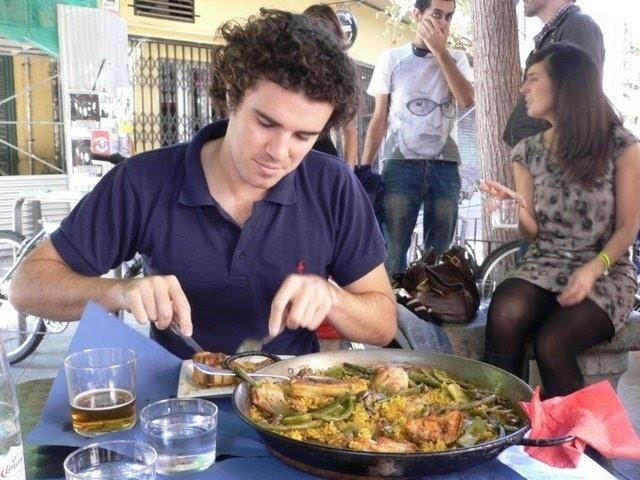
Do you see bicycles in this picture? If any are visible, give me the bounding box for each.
[409,172,521,303]
[0,150,145,366]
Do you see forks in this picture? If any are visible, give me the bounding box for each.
[235,322,286,356]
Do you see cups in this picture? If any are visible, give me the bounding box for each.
[63,345,138,439]
[62,438,158,480]
[137,397,220,477]
[489,199,521,232]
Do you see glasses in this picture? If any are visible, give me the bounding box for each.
[407,98,456,118]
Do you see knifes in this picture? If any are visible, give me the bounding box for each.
[166,320,204,353]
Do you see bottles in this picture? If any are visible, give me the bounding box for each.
[1,338,28,480]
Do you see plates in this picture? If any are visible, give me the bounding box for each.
[175,352,295,398]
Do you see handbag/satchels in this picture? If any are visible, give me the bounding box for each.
[393,246,480,323]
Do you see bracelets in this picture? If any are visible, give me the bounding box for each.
[600,251,612,269]
[597,254,609,271]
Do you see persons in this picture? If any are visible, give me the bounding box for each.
[8,7,399,366]
[501,0,608,256]
[301,3,358,167]
[478,40,640,399]
[355,0,477,288]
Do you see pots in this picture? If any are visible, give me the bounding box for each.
[224,348,579,480]
